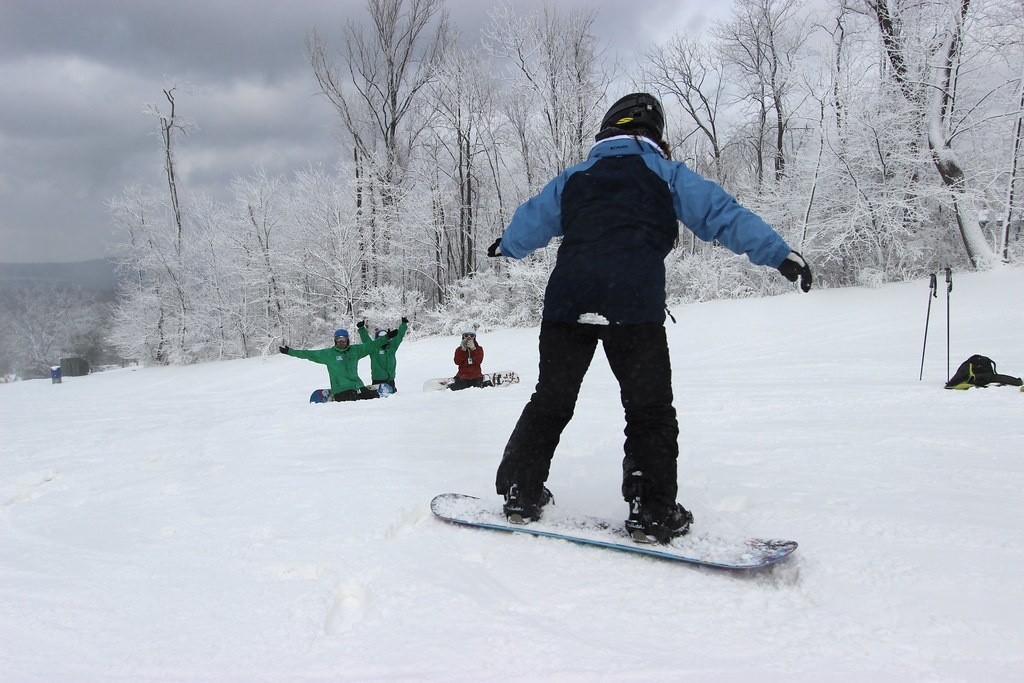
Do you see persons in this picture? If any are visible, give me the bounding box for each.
[485,91,813,546]
[447,328,495,391]
[358,317,410,393]
[279,328,399,404]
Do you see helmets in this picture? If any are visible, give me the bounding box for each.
[334,329,348,337]
[600,93,665,138]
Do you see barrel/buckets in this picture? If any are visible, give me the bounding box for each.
[51,366,62,383]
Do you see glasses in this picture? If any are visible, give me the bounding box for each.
[334,337,349,343]
[463,333,475,337]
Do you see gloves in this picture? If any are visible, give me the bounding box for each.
[279,346,289,354]
[467,340,476,351]
[387,328,398,338]
[461,339,467,351]
[488,238,502,257]
[357,320,365,328]
[402,317,410,323]
[778,250,813,293]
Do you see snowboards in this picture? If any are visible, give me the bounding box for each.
[309,382,396,404]
[422,369,520,392]
[428,491,798,572]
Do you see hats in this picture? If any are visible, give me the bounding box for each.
[462,327,476,335]
[374,328,387,337]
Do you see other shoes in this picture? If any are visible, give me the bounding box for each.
[367,385,376,390]
[328,395,334,402]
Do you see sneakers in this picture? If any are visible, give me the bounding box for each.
[503,484,555,522]
[625,500,693,536]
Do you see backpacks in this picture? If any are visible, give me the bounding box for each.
[945,355,1023,390]
